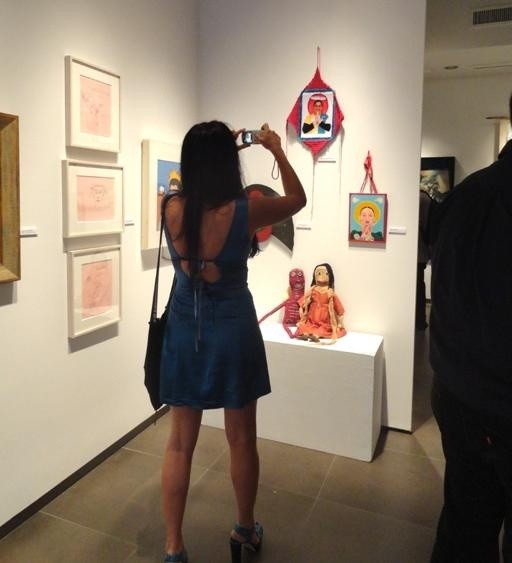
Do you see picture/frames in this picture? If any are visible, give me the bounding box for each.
[298,89,335,142]
[348,193,387,242]
[420,156,456,193]
[68,246,122,338]
[65,55,121,154]
[62,160,125,239]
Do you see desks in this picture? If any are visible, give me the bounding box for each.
[201,320,385,462]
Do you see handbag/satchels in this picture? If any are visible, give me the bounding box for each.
[143,314,167,411]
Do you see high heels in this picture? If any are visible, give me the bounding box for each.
[229,522,264,561]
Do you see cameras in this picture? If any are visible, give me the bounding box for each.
[242,131,264,144]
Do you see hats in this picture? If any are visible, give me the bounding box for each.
[353,200,381,225]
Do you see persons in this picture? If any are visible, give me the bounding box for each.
[297,262,346,342]
[422,92,512,563]
[350,206,383,241]
[302,100,331,134]
[144,117,308,563]
[415,189,437,330]
